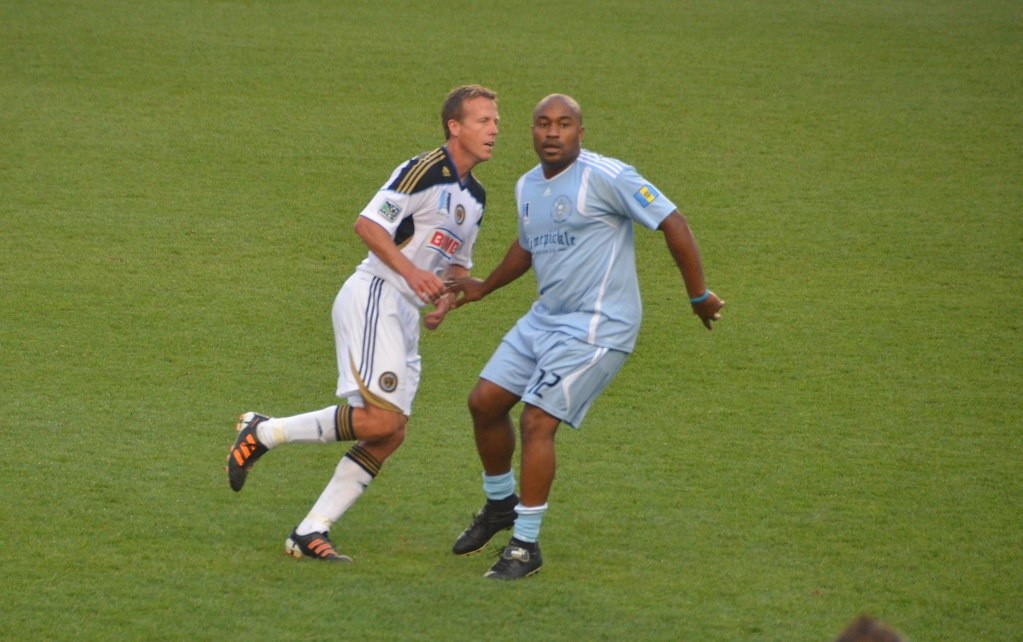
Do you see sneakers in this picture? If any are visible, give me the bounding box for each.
[223,412,271,492]
[284,525,353,562]
[483,536,543,580]
[453,493,520,554]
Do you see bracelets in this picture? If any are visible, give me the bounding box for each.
[689,290,709,303]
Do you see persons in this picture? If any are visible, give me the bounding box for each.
[225,85,500,562]
[443,94,727,581]
